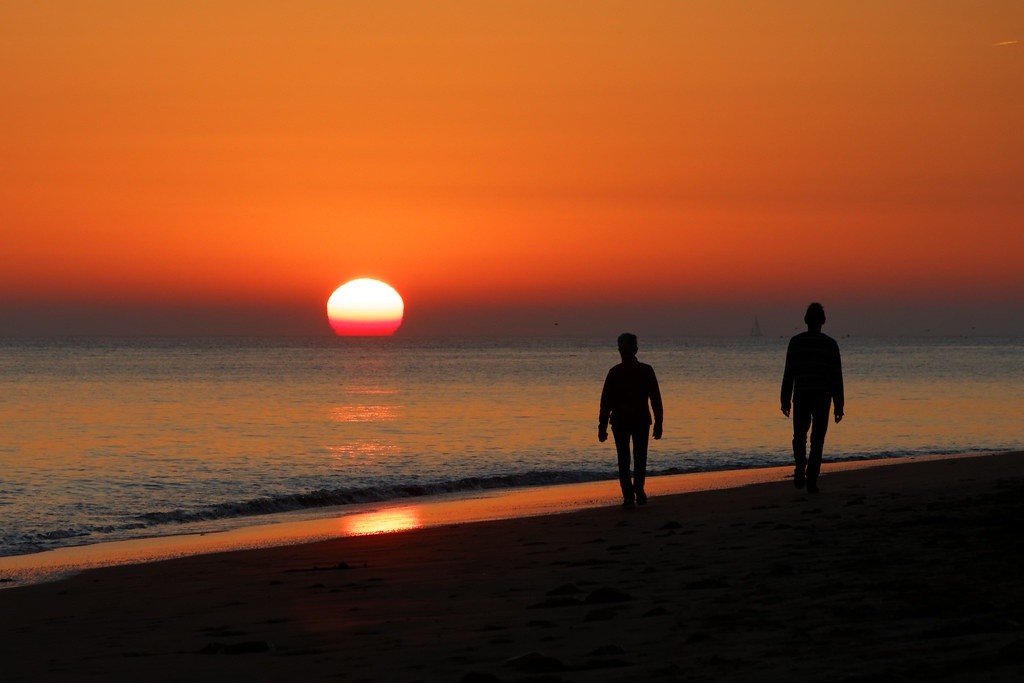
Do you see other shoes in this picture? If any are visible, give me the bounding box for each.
[632,484,647,505]
[794,469,805,490]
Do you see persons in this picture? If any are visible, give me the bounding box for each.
[598,332,664,509]
[780,302,845,491]
